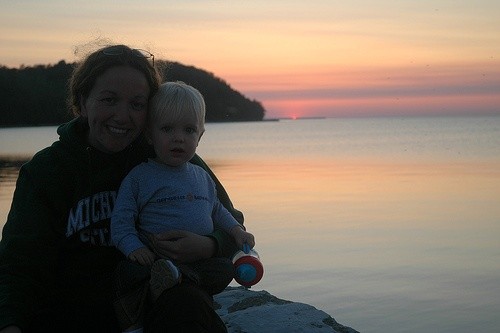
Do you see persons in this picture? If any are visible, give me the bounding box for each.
[0,45,247,333]
[111,80,255,333]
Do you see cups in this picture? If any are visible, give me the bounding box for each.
[230,241,266,289]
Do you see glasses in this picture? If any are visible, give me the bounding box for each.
[103,47,155,68]
